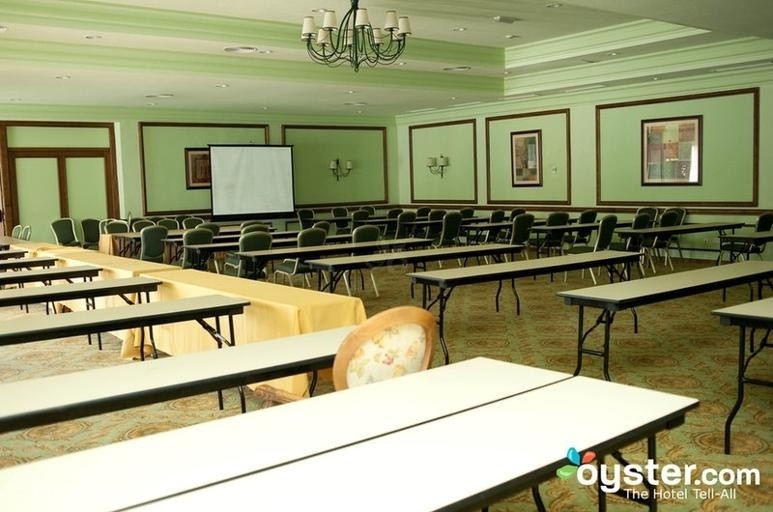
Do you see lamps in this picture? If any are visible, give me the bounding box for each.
[426,155,448,179]
[300,0,413,73]
[330,159,353,181]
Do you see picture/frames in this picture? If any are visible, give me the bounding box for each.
[185,148,211,189]
[641,115,703,186]
[510,129,543,187]
